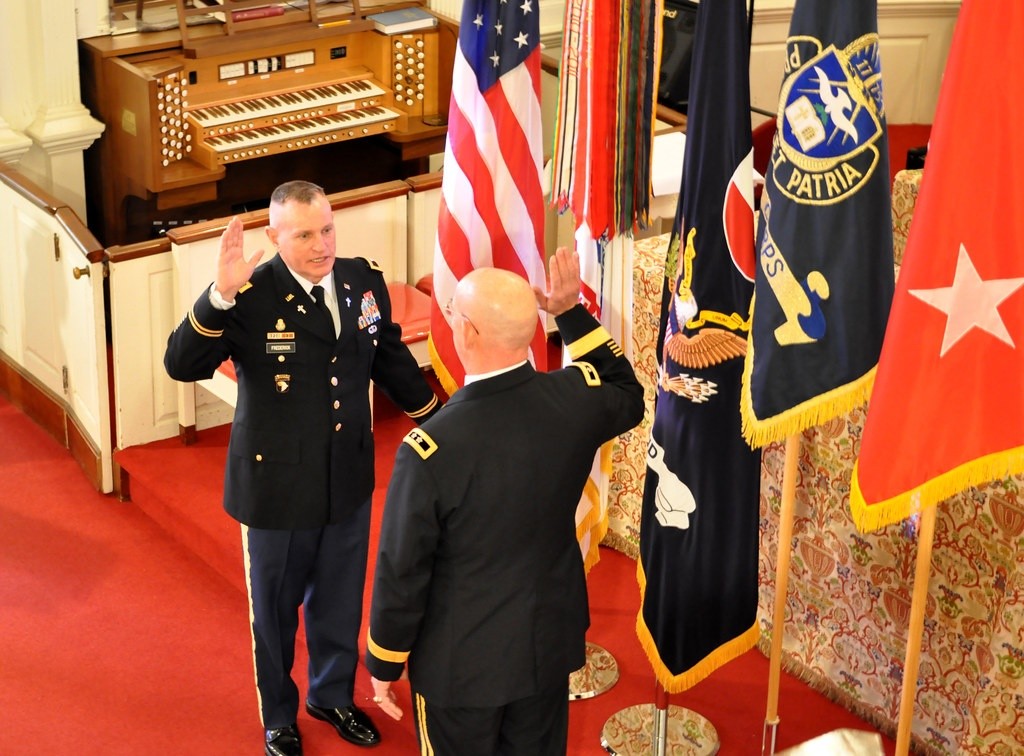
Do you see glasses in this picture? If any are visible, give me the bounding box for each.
[444,299,480,340]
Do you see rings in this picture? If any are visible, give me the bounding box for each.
[372,696,382,704]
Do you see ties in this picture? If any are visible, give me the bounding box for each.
[311,284,336,339]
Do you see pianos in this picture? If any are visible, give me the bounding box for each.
[189,77,401,165]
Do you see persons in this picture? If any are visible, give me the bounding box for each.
[362,247,645,756]
[163,180,446,756]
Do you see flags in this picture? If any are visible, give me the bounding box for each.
[847,0,1024,535]
[637,1,762,695]
[428,0,549,397]
[740,0,896,451]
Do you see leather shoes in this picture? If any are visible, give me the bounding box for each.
[263,725,301,756]
[306,699,381,746]
